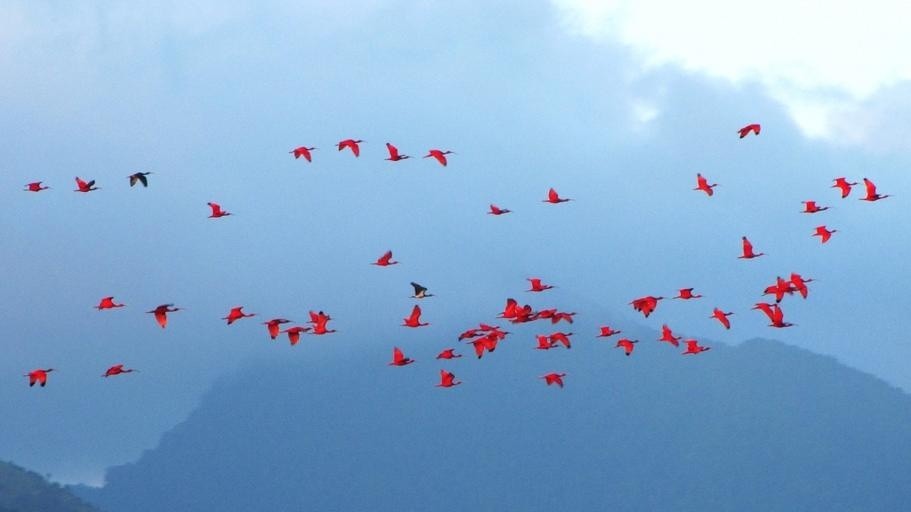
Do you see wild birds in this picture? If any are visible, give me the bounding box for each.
[734,236,765,260]
[739,123,761,139]
[92,293,182,330]
[595,271,815,359]
[220,305,335,346]
[372,250,399,269]
[286,138,456,167]
[693,173,719,197]
[486,187,570,216]
[393,277,577,389]
[104,359,135,381]
[22,366,55,389]
[800,167,893,244]
[22,166,152,193]
[206,201,233,220]
